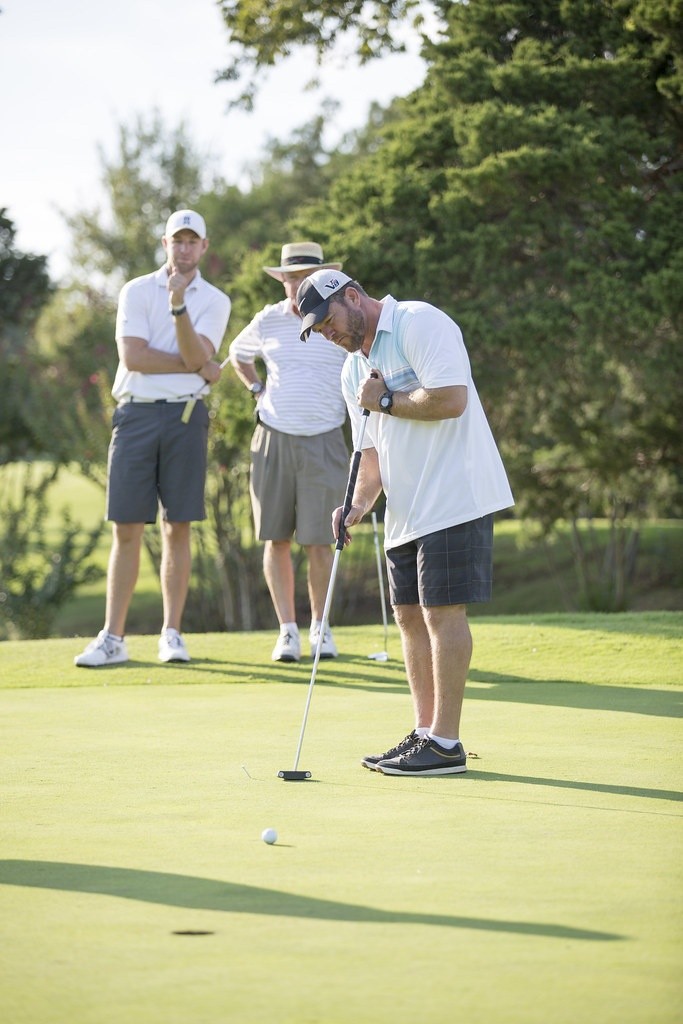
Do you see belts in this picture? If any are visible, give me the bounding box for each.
[116,392,203,405]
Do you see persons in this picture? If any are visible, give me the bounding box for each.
[74,210,231,667]
[296,269,515,775]
[230,242,350,662]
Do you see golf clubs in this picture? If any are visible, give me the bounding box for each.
[276,371,379,780]
[180,356,230,425]
[365,504,391,662]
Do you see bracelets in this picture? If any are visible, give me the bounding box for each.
[171,305,186,316]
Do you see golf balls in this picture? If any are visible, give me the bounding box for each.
[261,828,278,844]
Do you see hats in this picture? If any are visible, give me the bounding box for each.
[164,210,210,241]
[260,241,344,284]
[295,267,353,342]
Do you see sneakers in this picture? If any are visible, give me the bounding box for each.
[306,628,339,660]
[157,629,192,664]
[373,735,467,776]
[272,628,302,665]
[360,729,423,771]
[74,634,132,668]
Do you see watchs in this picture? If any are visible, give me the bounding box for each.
[250,383,263,395]
[379,391,395,415]
